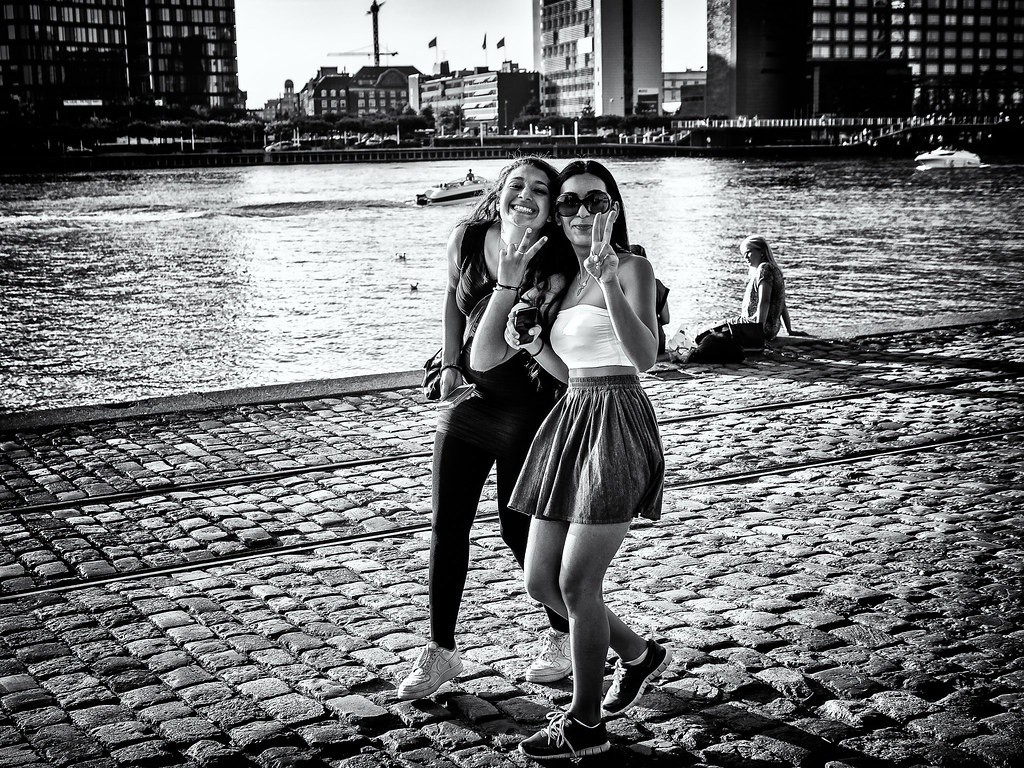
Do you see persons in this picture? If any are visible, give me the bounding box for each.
[696,235,810,346]
[466,169,474,181]
[398,158,573,700]
[503,161,672,759]
[629,245,670,355]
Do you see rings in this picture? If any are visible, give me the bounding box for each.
[517,250,524,255]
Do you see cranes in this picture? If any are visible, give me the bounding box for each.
[325,37,399,67]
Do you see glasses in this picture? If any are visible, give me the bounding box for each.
[555,189,613,218]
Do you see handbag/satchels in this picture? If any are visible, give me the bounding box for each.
[421,347,442,400]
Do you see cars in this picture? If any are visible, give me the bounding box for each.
[265,140,294,152]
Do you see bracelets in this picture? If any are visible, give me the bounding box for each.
[531,340,544,357]
[440,364,462,373]
[493,282,521,292]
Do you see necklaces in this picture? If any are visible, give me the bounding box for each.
[576,272,591,296]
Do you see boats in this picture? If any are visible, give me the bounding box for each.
[914,145,991,171]
[413,177,495,207]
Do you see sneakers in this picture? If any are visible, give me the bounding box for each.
[518,711,610,760]
[526,626,573,682]
[601,638,672,717]
[397,642,464,701]
